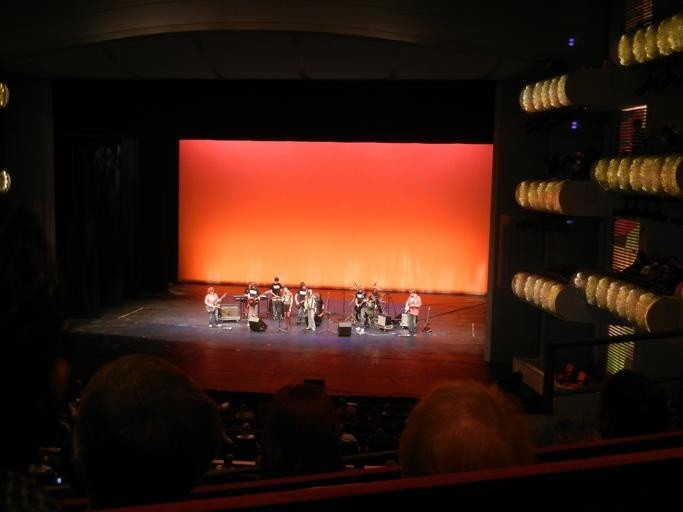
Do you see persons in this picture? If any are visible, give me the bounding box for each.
[244,282,252,295]
[354,284,368,326]
[205,287,223,328]
[403,289,422,337]
[282,286,293,320]
[268,276,282,314]
[219,399,257,462]
[369,288,385,315]
[66,355,233,508]
[398,381,535,478]
[572,365,683,455]
[335,398,413,463]
[256,385,342,479]
[30,448,59,490]
[304,289,317,331]
[294,282,309,325]
[247,282,260,327]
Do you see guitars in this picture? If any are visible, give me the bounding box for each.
[206,293,227,313]
[405,295,418,313]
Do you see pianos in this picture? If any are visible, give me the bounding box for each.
[233,295,266,301]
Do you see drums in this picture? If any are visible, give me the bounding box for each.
[272,298,284,320]
